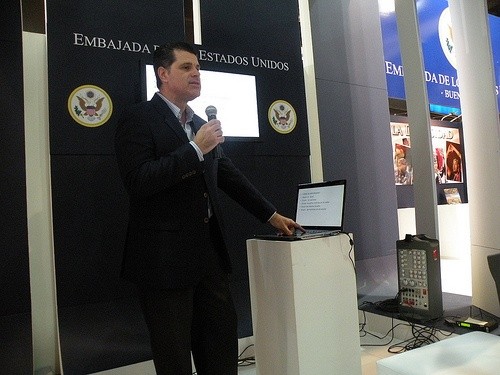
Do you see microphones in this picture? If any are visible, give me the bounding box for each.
[205,105,222,160]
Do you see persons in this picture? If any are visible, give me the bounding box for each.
[436,148,446,183]
[443,143,462,181]
[114,41,306,375]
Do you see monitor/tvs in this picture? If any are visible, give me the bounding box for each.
[140,56,264,143]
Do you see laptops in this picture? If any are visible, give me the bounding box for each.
[254,179,347,241]
